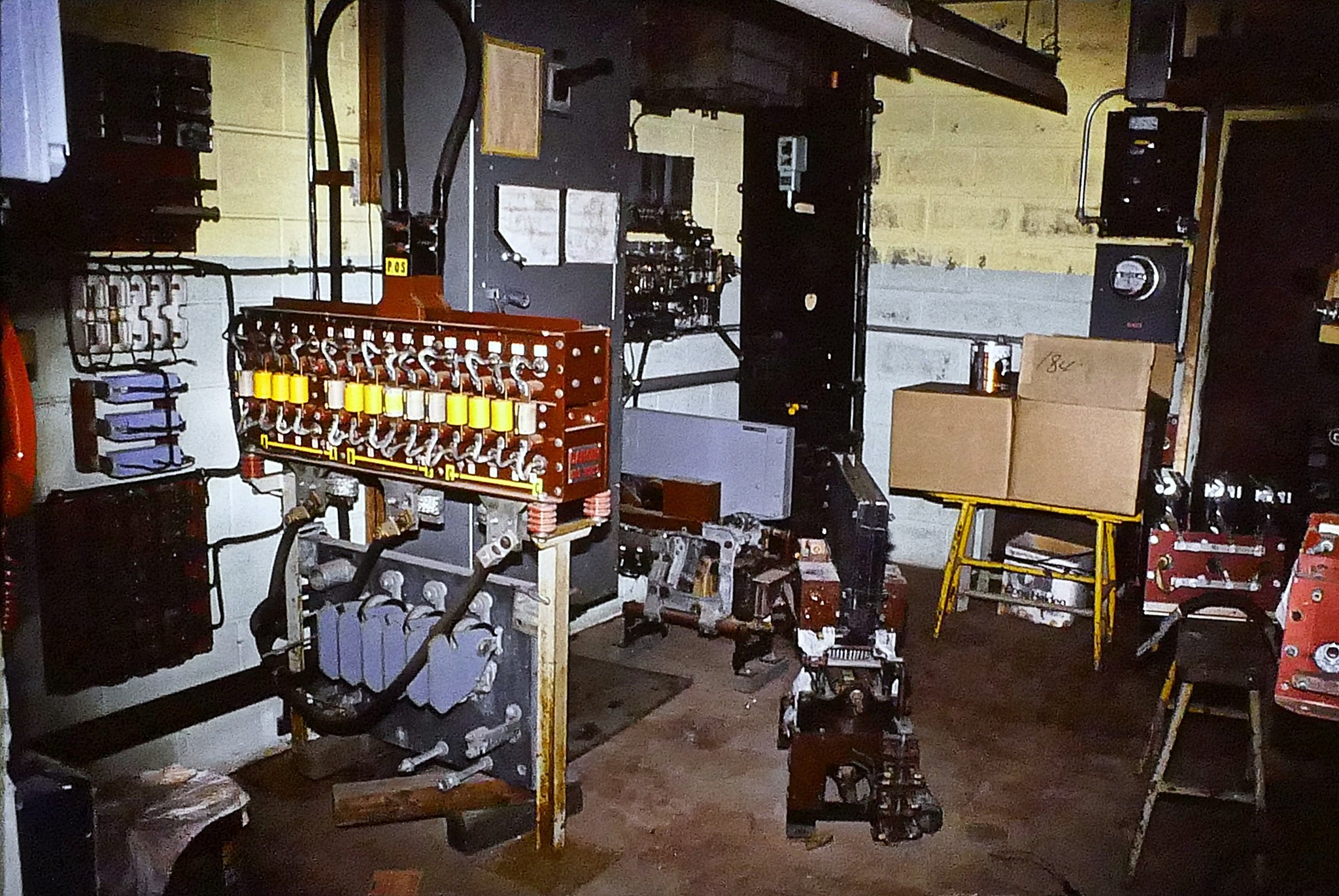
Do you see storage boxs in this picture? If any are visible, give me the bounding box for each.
[888,333,1178,516]
[998,531,1096,628]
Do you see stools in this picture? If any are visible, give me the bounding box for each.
[1124,619,1270,877]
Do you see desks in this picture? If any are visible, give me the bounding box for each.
[926,491,1146,669]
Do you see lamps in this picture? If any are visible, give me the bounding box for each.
[910,0,1070,108]
